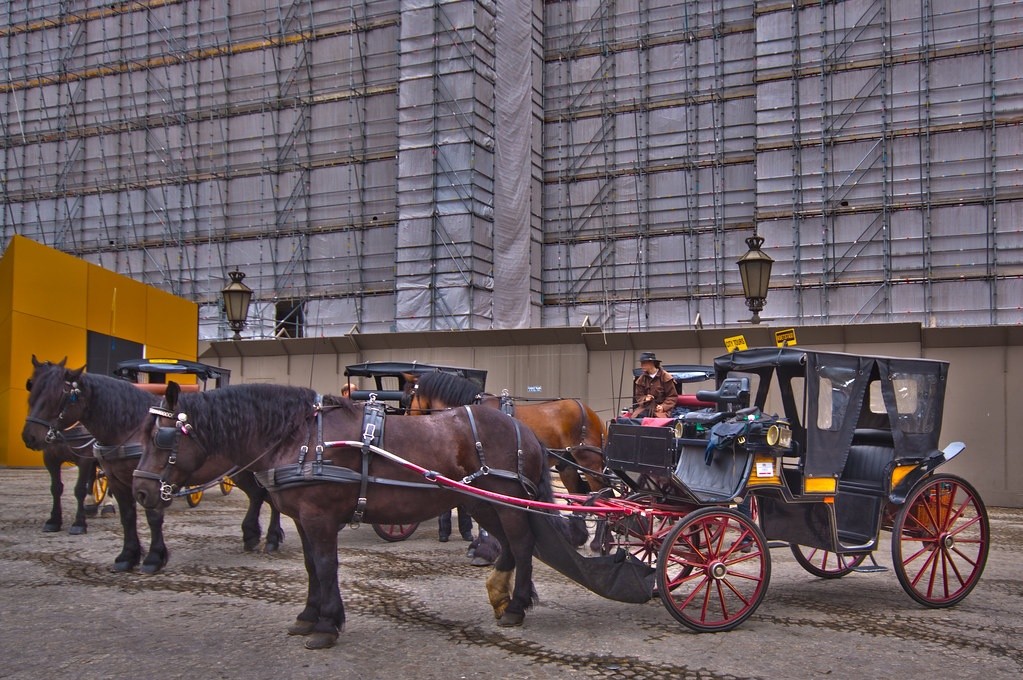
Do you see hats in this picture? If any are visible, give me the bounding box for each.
[635,352,663,362]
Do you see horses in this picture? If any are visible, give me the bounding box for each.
[21,353,283,573]
[130,371,576,649]
[401,371,616,500]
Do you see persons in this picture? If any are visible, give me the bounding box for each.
[341,382,358,398]
[633,352,678,417]
[738,489,753,552]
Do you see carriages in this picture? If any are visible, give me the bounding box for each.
[395,365,743,554]
[20,359,489,576]
[132,346,990,653]
[21,338,238,536]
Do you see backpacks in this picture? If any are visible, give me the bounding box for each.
[704,405,775,466]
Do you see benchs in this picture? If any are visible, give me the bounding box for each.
[834,428,895,539]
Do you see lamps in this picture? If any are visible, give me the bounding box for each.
[736,225,776,324]
[220,265,255,340]
